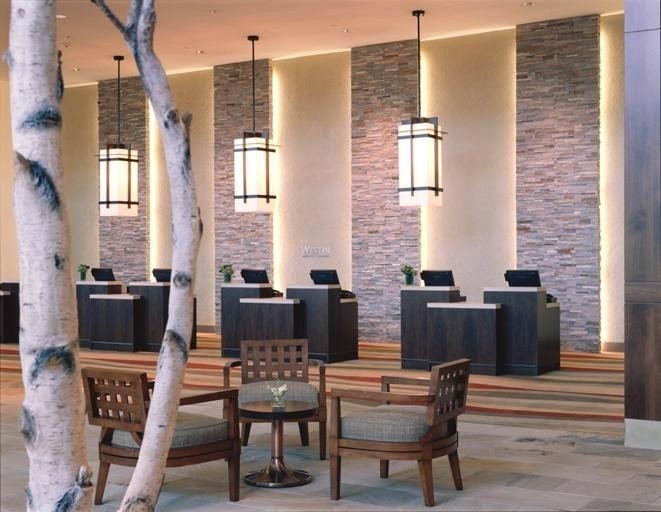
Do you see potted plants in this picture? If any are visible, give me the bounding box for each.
[400,265,418,285]
[219,264,234,282]
[77,264,90,280]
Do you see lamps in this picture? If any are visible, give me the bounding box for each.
[396,8,445,209]
[99,54,140,218]
[232,34,282,215]
